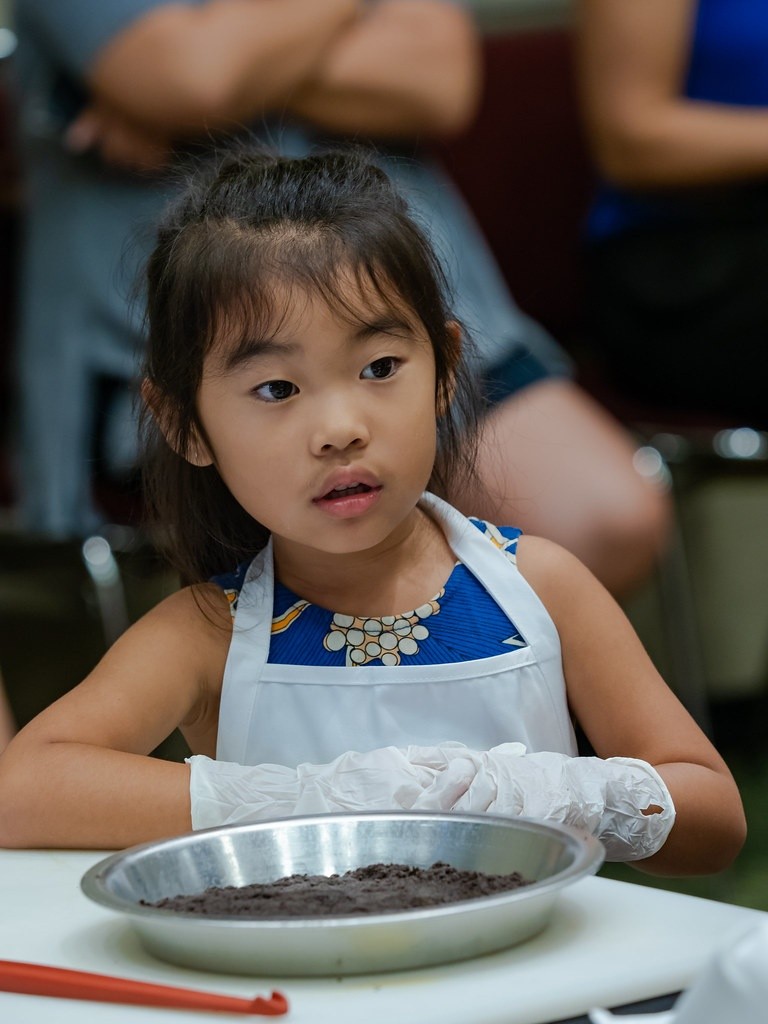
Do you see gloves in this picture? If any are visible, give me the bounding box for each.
[185,742,676,863]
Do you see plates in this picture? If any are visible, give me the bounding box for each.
[80,809,606,976]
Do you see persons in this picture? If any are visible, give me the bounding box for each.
[0,154,747,873]
[581,0,768,427]
[15,0,675,598]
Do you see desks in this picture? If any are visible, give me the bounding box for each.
[0,848,768,1024]
